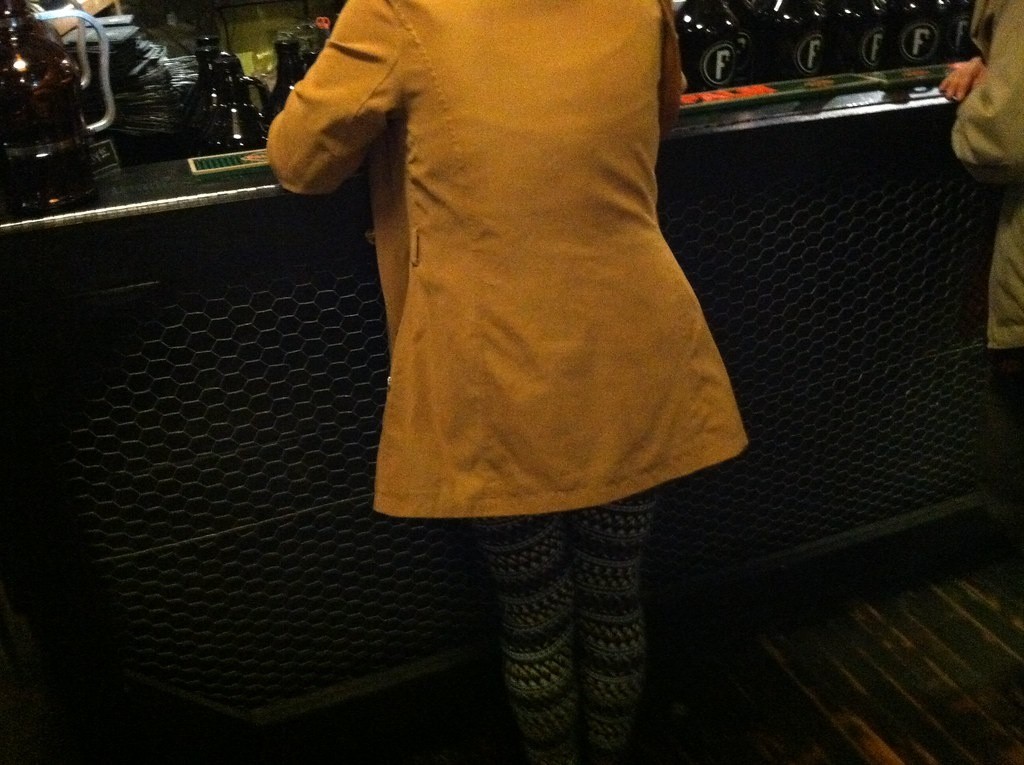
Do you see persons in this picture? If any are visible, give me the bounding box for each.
[268,1,749,764]
[941,0,1024,561]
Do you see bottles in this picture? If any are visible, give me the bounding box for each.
[0,0,116,215]
[180,36,322,157]
[675,0,977,93]
